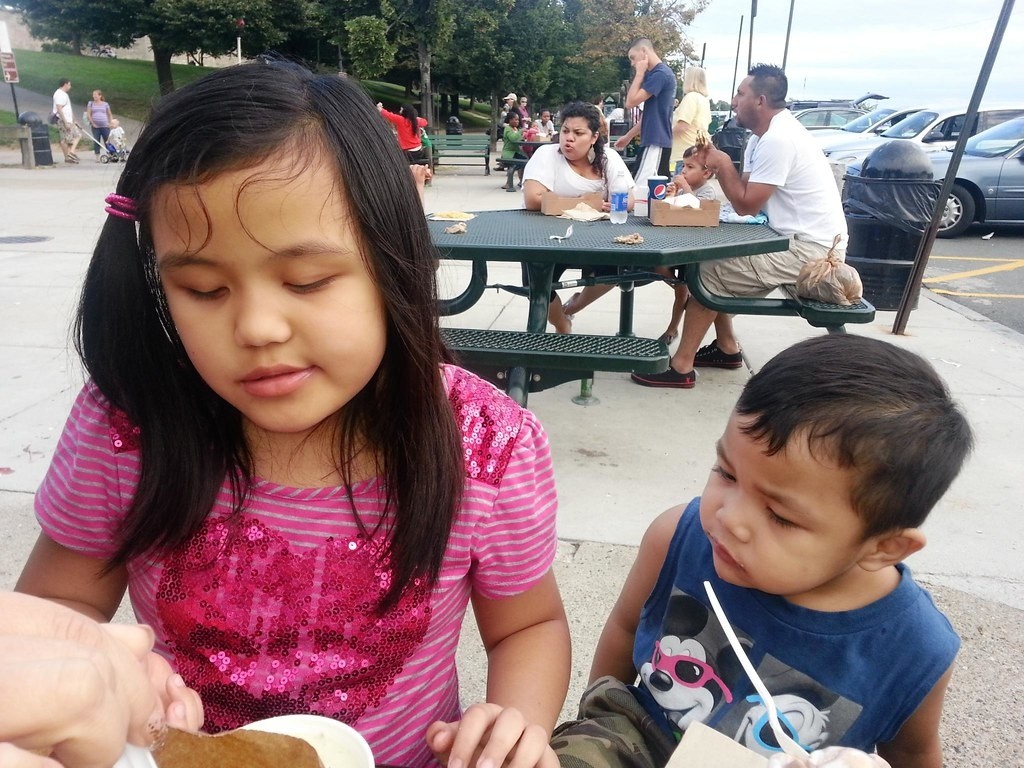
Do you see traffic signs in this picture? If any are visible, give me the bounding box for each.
[2,70,19,83]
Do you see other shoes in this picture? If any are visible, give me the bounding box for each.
[67,152,80,160]
[518,182,522,187]
[493,167,504,171]
[657,330,678,345]
[65,159,79,164]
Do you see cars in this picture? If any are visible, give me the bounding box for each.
[808,104,938,156]
[842,108,1024,239]
[822,104,1024,199]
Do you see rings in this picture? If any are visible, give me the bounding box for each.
[705,165,708,168]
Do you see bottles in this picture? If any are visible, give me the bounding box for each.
[609,170,628,224]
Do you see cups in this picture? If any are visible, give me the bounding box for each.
[647,176,669,220]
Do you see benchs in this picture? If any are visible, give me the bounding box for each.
[428,135,492,176]
[440,326,671,390]
[778,281,876,335]
[498,156,529,170]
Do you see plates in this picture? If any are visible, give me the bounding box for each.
[237,714,375,768]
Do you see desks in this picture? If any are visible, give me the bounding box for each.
[424,211,845,410]
[502,141,554,191]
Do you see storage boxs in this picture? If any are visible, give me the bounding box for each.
[651,197,720,227]
[540,191,603,215]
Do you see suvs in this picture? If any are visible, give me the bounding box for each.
[782,93,892,119]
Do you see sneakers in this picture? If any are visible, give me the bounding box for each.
[631,364,696,388]
[693,339,742,368]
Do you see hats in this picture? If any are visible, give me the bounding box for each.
[502,93,518,101]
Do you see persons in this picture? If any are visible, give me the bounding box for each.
[663,145,716,201]
[501,92,554,187]
[86,90,113,164]
[15,62,571,768]
[613,39,677,188]
[0,590,153,768]
[630,64,850,388]
[52,79,82,164]
[377,102,433,191]
[667,67,712,181]
[108,119,125,162]
[520,102,634,211]
[548,333,976,768]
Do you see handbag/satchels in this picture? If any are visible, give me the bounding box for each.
[797,233,864,305]
[83,101,93,127]
[48,112,59,124]
[420,128,432,147]
